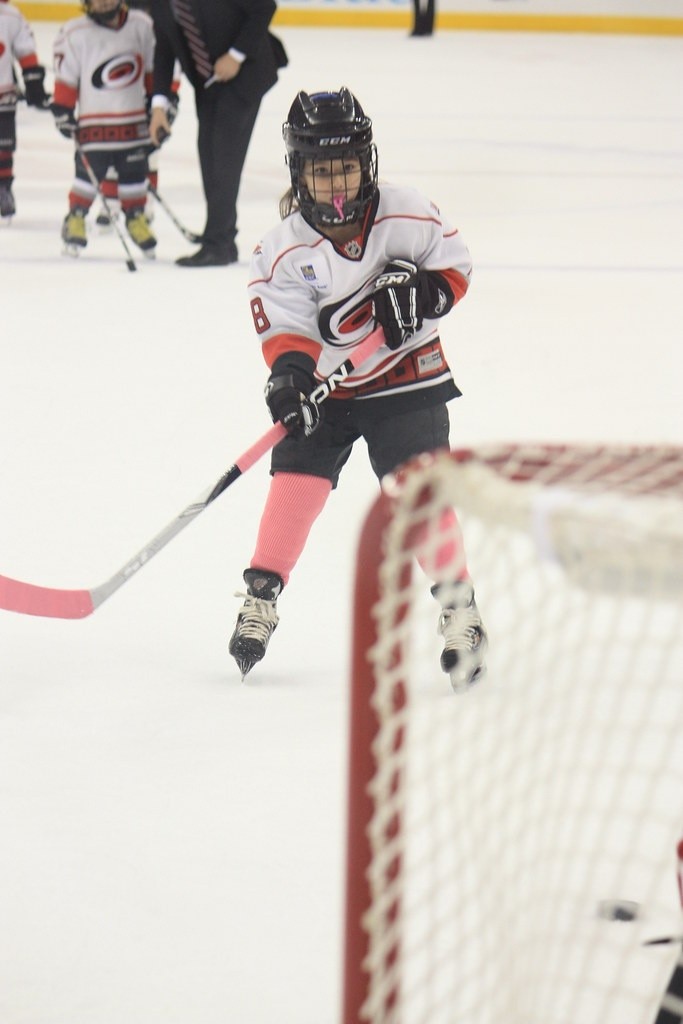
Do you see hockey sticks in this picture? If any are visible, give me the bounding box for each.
[0,326,387,620]
[147,186,241,245]
[69,131,139,273]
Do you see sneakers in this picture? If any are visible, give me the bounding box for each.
[431,581,487,695]
[229,568,284,683]
[122,207,157,258]
[61,206,88,258]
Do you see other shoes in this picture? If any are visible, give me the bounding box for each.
[0,177,16,215]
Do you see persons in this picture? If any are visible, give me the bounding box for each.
[148,0,287,267]
[228,86,487,694]
[52,0,180,260]
[0,0,48,215]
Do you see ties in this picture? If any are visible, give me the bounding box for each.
[172,0,214,78]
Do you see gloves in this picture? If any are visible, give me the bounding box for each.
[146,92,179,129]
[264,351,325,438]
[50,104,78,137]
[22,66,47,108]
[372,259,423,351]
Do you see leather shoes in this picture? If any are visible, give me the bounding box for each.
[176,239,237,267]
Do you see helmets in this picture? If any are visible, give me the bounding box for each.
[281,88,379,228]
